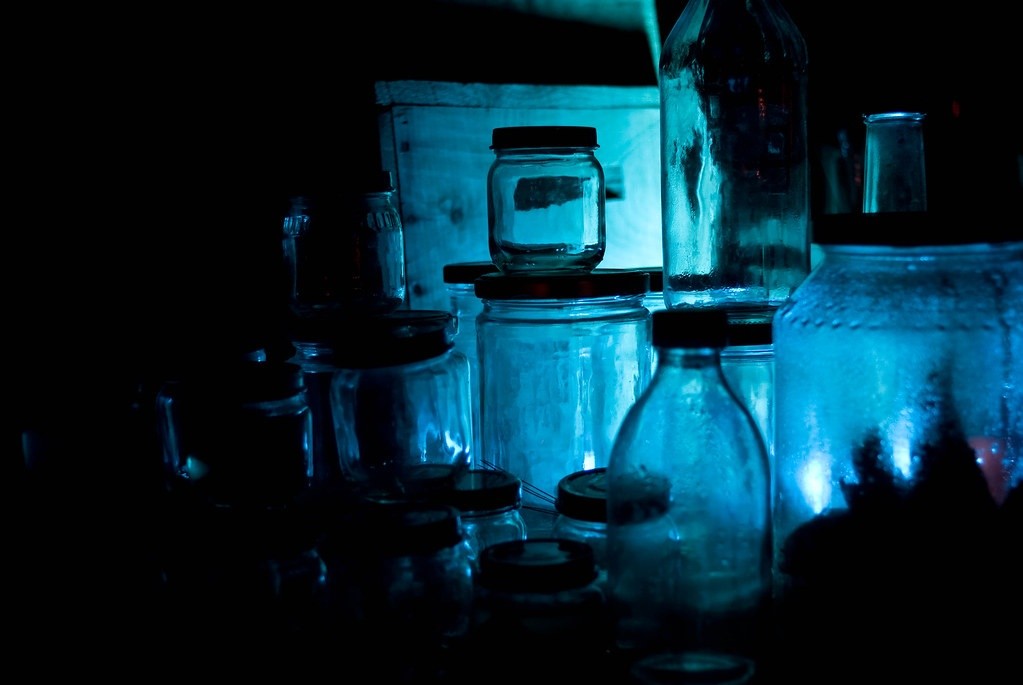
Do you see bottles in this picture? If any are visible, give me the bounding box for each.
[659,0,815,308]
[601,304,775,680]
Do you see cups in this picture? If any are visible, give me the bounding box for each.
[486,123,607,277]
[155,283,667,685]
[858,104,931,213]
